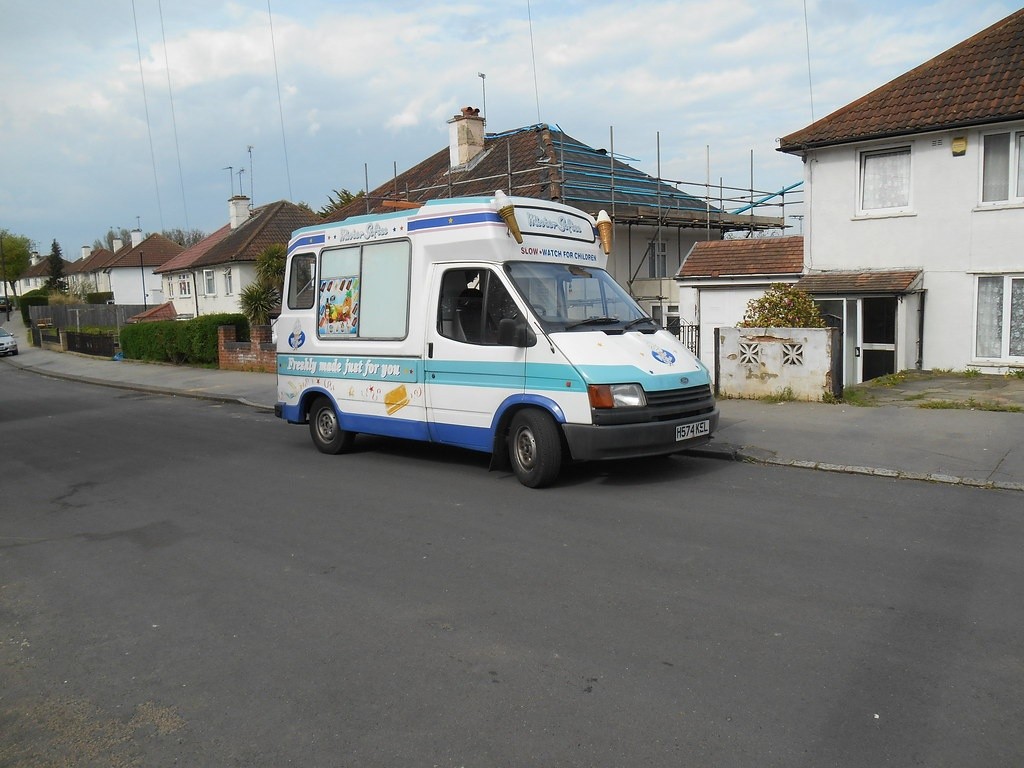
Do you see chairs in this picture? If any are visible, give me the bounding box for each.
[451,289,498,345]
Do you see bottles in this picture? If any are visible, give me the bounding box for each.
[330,295,335,305]
[326,298,329,320]
[320,282,326,292]
[320,316,325,327]
[327,281,333,292]
[346,279,352,290]
[339,280,345,291]
[352,317,357,326]
[343,296,351,319]
[352,302,358,314]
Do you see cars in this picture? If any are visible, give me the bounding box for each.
[0,327,19,357]
[0,297,13,312]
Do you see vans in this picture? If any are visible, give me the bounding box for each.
[272,188,721,489]
